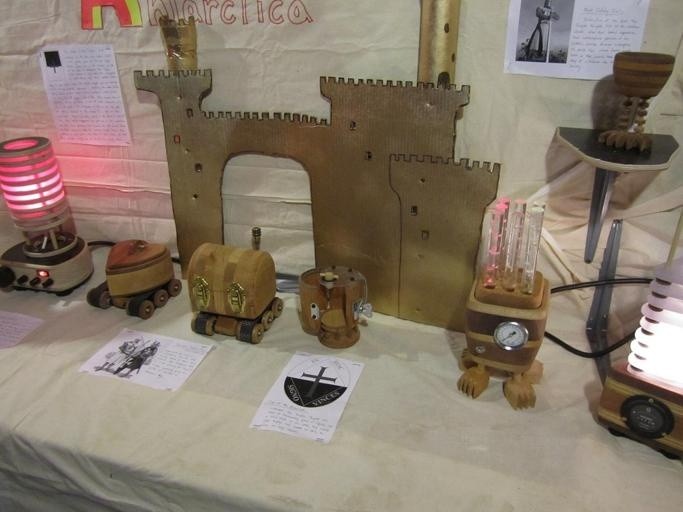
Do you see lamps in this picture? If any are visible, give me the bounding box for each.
[0,136,95,297]
[595,260,683,459]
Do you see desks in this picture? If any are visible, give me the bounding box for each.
[0,244,683,512]
